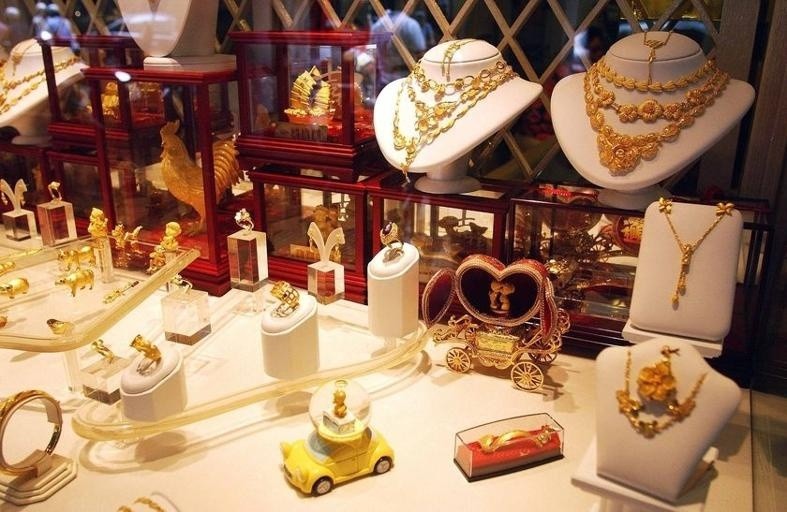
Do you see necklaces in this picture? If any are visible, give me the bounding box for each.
[393,36,518,175]
[0,40,83,117]
[584,30,733,176]
[659,198,735,306]
[615,348,709,438]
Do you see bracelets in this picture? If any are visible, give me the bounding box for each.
[0,389,63,474]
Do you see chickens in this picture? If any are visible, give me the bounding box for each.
[159,120,241,239]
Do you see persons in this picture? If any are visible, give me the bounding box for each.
[316,0,436,105]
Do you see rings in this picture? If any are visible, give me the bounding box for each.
[177,278,193,289]
[234,209,254,229]
[131,334,161,361]
[92,340,114,359]
[272,281,299,306]
[380,221,404,251]
[47,318,73,334]
